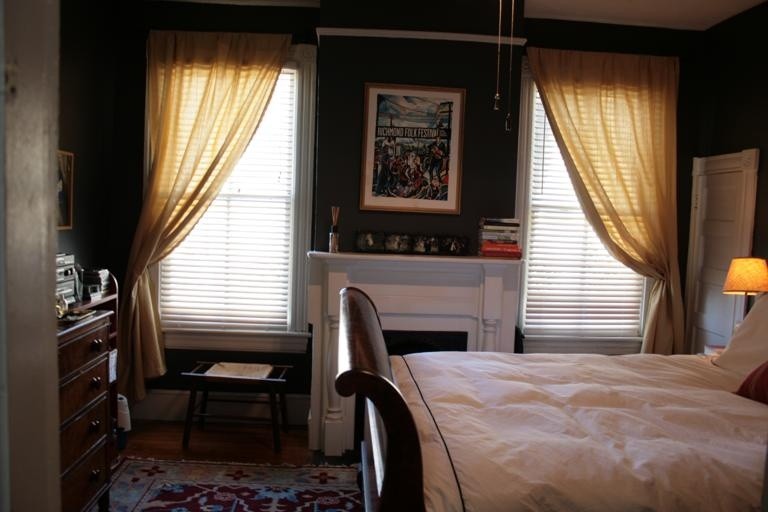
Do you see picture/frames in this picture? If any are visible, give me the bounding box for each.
[357,80,464,218]
[54,146,76,234]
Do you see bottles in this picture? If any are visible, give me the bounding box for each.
[328,224,341,253]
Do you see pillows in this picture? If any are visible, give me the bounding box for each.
[711,292,768,376]
[730,357,767,407]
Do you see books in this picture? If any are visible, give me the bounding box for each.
[702,344,726,357]
[469,215,522,258]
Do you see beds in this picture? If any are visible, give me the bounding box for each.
[331,286,767,512]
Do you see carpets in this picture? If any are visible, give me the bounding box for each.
[76,453,379,512]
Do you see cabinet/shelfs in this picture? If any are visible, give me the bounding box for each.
[68,270,121,472]
[51,308,116,512]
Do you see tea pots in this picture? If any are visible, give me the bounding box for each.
[356,230,471,256]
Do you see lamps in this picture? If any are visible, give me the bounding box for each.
[487,0,517,137]
[721,255,767,319]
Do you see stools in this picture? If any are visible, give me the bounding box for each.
[178,359,294,454]
